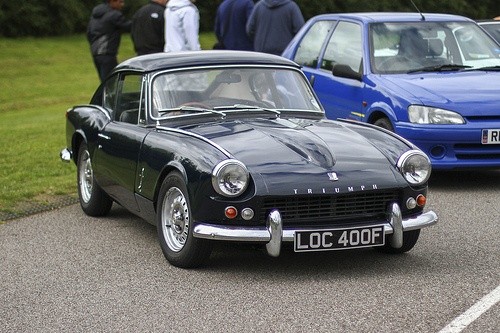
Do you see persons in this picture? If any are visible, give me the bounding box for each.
[87,0,132,93]
[163,0,209,91]
[130,0,173,91]
[212,0,305,102]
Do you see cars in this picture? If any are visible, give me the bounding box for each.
[427,20,500,71]
[58,49,439,270]
[278,12,500,179]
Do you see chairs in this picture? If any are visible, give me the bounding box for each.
[120,108,145,124]
[423,39,445,63]
